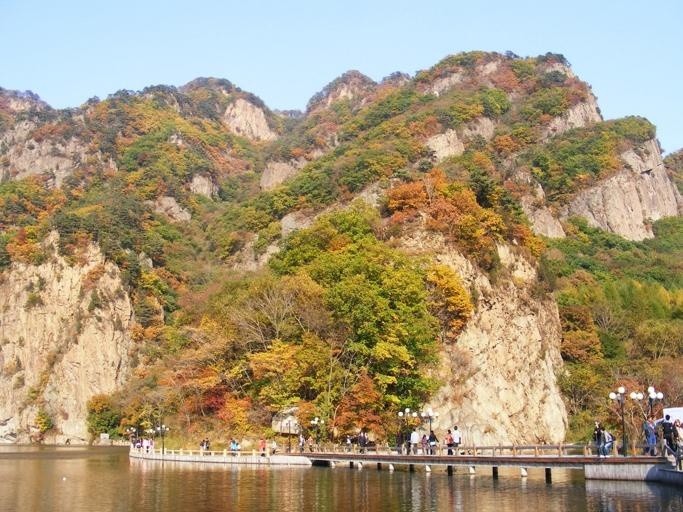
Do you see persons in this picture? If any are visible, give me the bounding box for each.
[396,428,439,455]
[131,436,154,454]
[345,430,369,454]
[593,421,613,456]
[643,414,683,465]
[445,425,460,456]
[227,438,241,456]
[259,435,276,456]
[308,435,314,453]
[299,434,305,453]
[65,439,71,445]
[200,438,210,451]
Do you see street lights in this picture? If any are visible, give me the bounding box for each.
[421,407,439,454]
[157,425,169,453]
[311,417,324,452]
[609,386,664,456]
[286,417,292,454]
[398,408,418,454]
